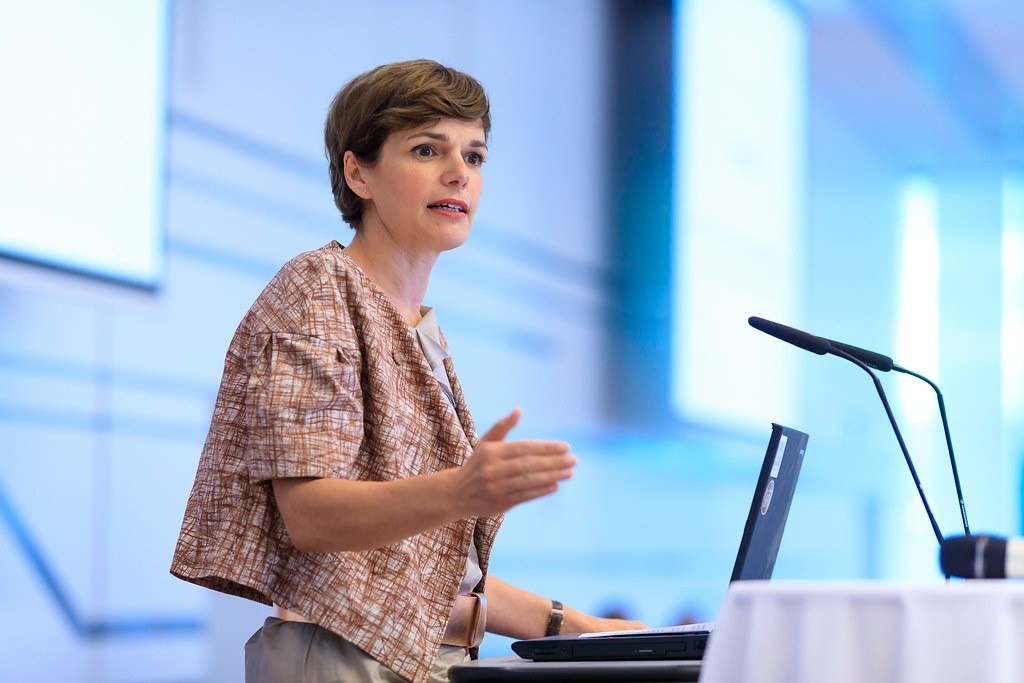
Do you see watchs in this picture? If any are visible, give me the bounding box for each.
[544,600,564,637]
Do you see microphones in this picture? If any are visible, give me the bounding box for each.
[749,316,1024,579]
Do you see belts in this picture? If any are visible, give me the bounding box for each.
[272,592,489,649]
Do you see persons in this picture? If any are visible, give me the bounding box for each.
[170,59,653,683]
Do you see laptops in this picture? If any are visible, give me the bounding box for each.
[511,421,809,662]
